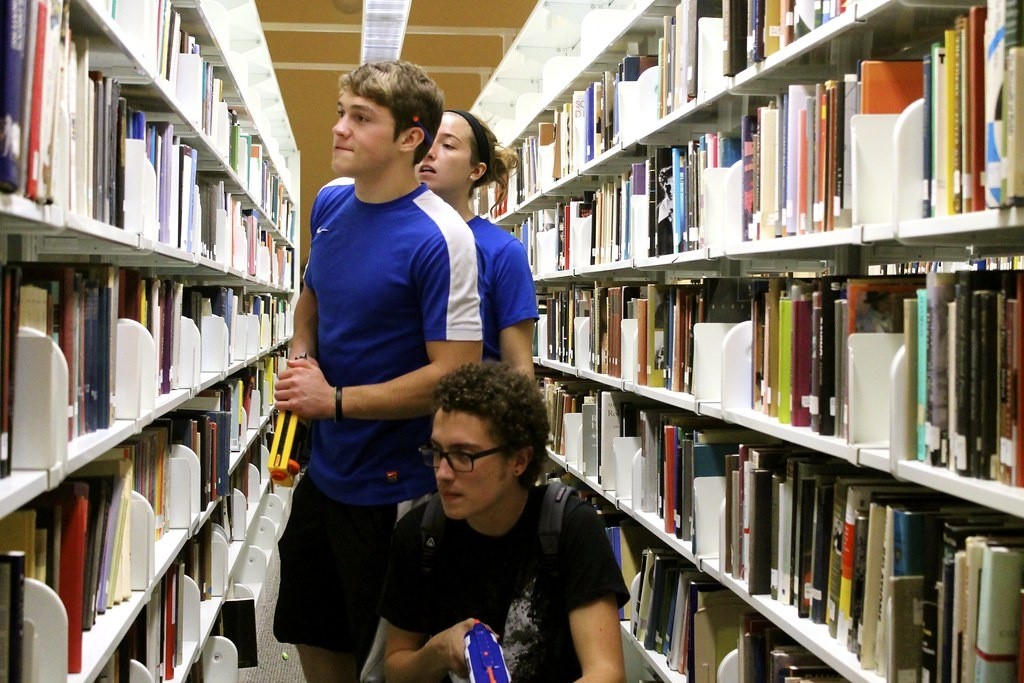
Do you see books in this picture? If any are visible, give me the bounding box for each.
[466,0,1024,683]
[0,0,314,683]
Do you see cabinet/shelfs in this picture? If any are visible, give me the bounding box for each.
[467,0,1024,683]
[0,0,301,683]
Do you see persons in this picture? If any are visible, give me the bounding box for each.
[415,109,540,381]
[272,59,482,683]
[377,358,628,683]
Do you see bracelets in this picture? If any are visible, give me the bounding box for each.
[336,386,343,424]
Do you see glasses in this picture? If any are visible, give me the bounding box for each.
[418,440,505,473]
